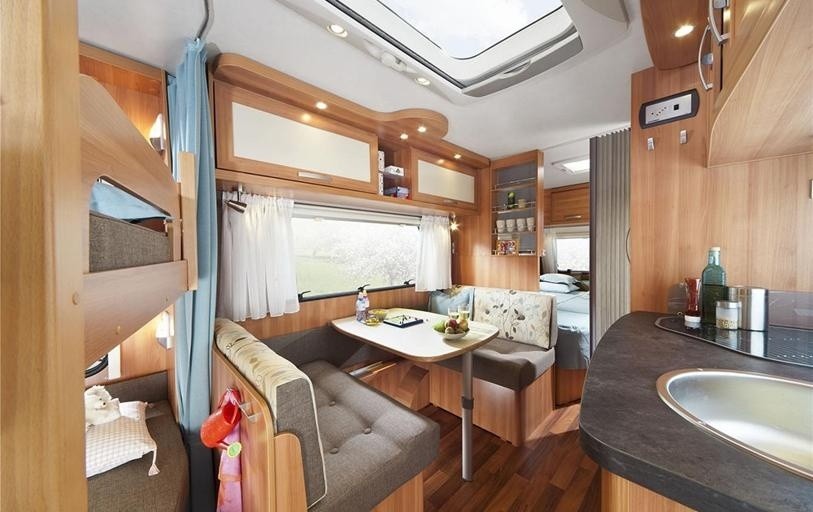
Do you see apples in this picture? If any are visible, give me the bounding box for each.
[434,319,468,333]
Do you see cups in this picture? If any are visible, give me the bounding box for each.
[518,198,526,208]
[447,303,470,320]
[738,286,770,332]
[495,217,536,233]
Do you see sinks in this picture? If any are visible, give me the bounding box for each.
[655,367,813,481]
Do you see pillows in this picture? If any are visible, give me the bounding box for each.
[539,273,580,294]
[83,398,160,483]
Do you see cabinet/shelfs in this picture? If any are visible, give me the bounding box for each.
[543,182,592,229]
[487,158,542,258]
[697,0,813,169]
[205,52,491,217]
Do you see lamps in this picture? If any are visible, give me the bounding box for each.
[146,109,168,160]
[152,309,174,354]
[223,182,248,215]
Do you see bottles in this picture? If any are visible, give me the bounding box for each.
[507,192,515,210]
[356,290,370,322]
[728,287,737,301]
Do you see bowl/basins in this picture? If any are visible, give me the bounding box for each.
[432,329,470,340]
[368,309,388,320]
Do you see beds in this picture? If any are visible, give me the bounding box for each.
[0,0,201,512]
[532,266,593,409]
